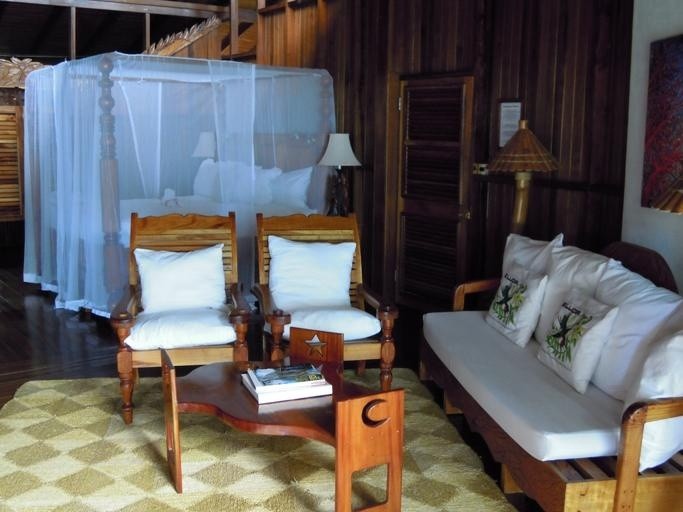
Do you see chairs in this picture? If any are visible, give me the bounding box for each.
[110,209,250,425]
[254,210,400,393]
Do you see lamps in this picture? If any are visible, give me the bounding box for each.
[190,130,216,159]
[316,131,361,216]
[484,117,561,241]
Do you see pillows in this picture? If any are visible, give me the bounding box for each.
[483,234,682,422]
[122,241,238,350]
[263,234,382,341]
[272,166,313,212]
[225,167,283,204]
[191,158,242,200]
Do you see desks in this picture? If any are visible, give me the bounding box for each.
[157,325,408,512]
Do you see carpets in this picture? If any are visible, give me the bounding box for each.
[0,360,520,510]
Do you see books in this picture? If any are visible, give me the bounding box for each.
[255,395,333,414]
[246,361,326,390]
[239,372,332,405]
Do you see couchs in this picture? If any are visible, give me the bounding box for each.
[419,233,683,511]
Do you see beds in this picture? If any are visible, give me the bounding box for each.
[21,53,343,338]
